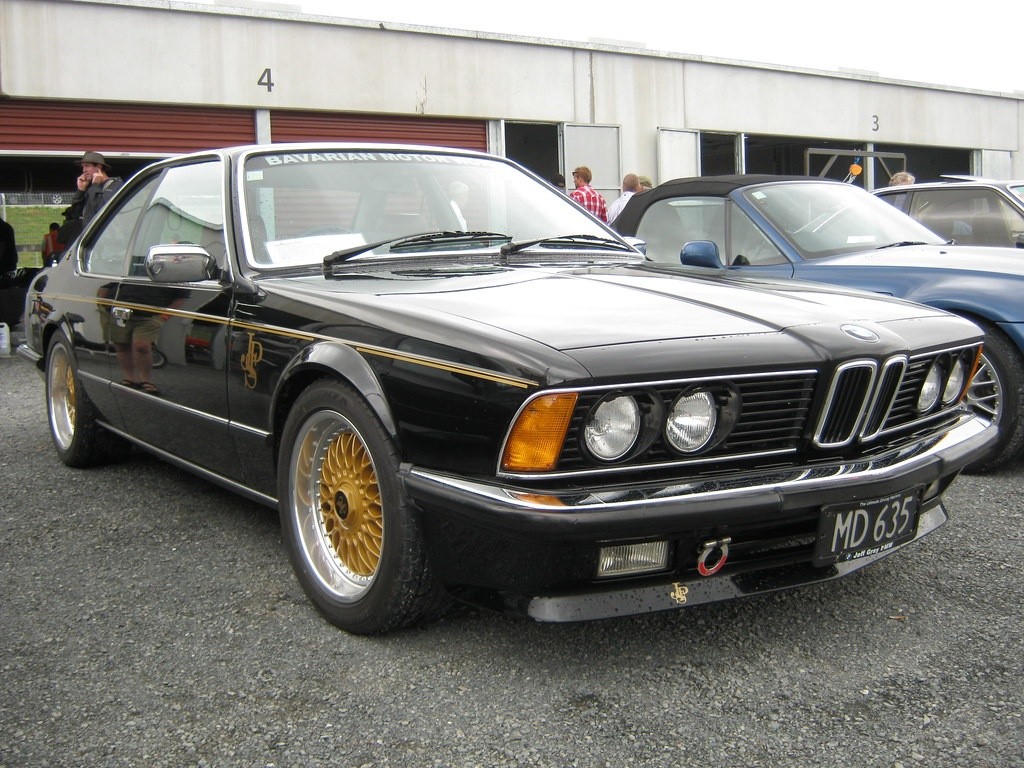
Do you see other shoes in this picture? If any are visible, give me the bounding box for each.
[121,378,138,388]
[141,381,159,396]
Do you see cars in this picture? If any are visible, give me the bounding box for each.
[15,140,1002,636]
[788,174,1024,261]
[40,193,73,204]
[604,174,1024,475]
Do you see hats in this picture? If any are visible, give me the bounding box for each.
[74,150,112,170]
[62,207,72,216]
[639,176,653,188]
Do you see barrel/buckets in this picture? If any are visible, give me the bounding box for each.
[0,322,11,355]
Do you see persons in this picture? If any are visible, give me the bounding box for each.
[56,207,84,250]
[0,218,18,276]
[608,174,654,226]
[96,281,191,395]
[889,172,916,186]
[41,222,65,267]
[31,275,119,352]
[570,167,609,222]
[552,173,565,188]
[71,151,124,230]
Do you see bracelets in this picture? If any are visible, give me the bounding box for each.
[162,315,168,319]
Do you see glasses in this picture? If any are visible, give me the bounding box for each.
[557,185,564,188]
[572,172,578,175]
